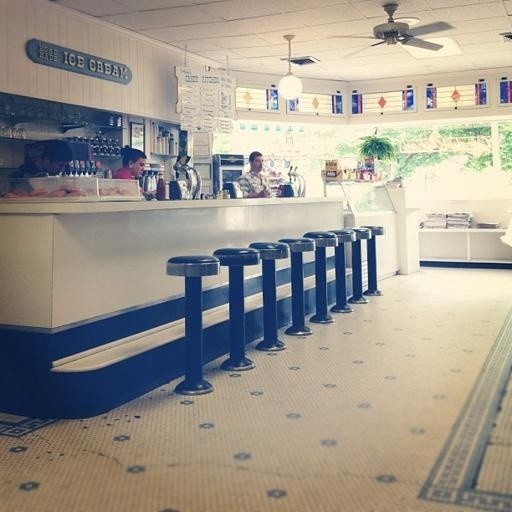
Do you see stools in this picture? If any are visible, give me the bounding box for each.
[327,229,358,314]
[212,246,263,373]
[347,227,372,305]
[279,237,317,336]
[359,225,385,297]
[165,254,220,397]
[245,242,290,353]
[302,230,340,325]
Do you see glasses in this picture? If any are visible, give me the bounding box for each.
[130,161,146,168]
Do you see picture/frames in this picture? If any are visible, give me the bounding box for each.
[130,121,145,156]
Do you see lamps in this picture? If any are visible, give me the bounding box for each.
[276,33,304,99]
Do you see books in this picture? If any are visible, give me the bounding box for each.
[425,212,499,229]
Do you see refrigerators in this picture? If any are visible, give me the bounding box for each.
[213,152,246,197]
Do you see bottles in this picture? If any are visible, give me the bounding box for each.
[75,134,121,157]
[153,122,178,155]
[137,171,166,201]
[105,168,113,179]
[68,159,97,177]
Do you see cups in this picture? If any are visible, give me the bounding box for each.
[0,126,28,140]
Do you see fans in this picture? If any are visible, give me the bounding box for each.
[325,0,454,54]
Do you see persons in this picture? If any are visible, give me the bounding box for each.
[7,140,72,192]
[113,145,157,196]
[236,151,275,198]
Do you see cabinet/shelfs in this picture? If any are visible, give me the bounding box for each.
[1,111,122,172]
[418,227,512,264]
[323,179,401,285]
